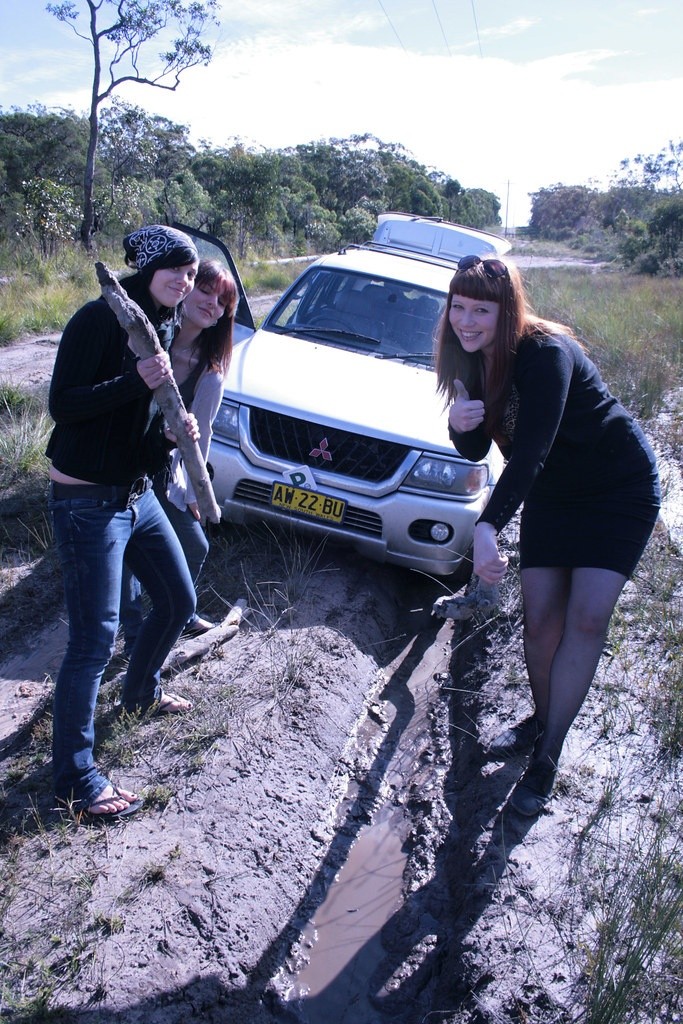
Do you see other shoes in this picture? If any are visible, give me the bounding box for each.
[489,716,544,758]
[509,732,559,817]
[182,623,219,636]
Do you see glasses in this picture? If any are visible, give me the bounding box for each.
[456,255,506,278]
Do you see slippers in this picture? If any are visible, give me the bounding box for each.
[152,693,192,715]
[53,779,143,819]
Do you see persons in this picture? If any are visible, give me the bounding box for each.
[434,255,661,815]
[43,223,198,818]
[115,259,240,660]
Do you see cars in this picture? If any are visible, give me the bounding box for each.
[169,211,515,578]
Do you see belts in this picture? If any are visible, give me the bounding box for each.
[53,477,146,510]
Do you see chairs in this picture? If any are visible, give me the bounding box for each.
[313,290,378,340]
[362,285,395,336]
